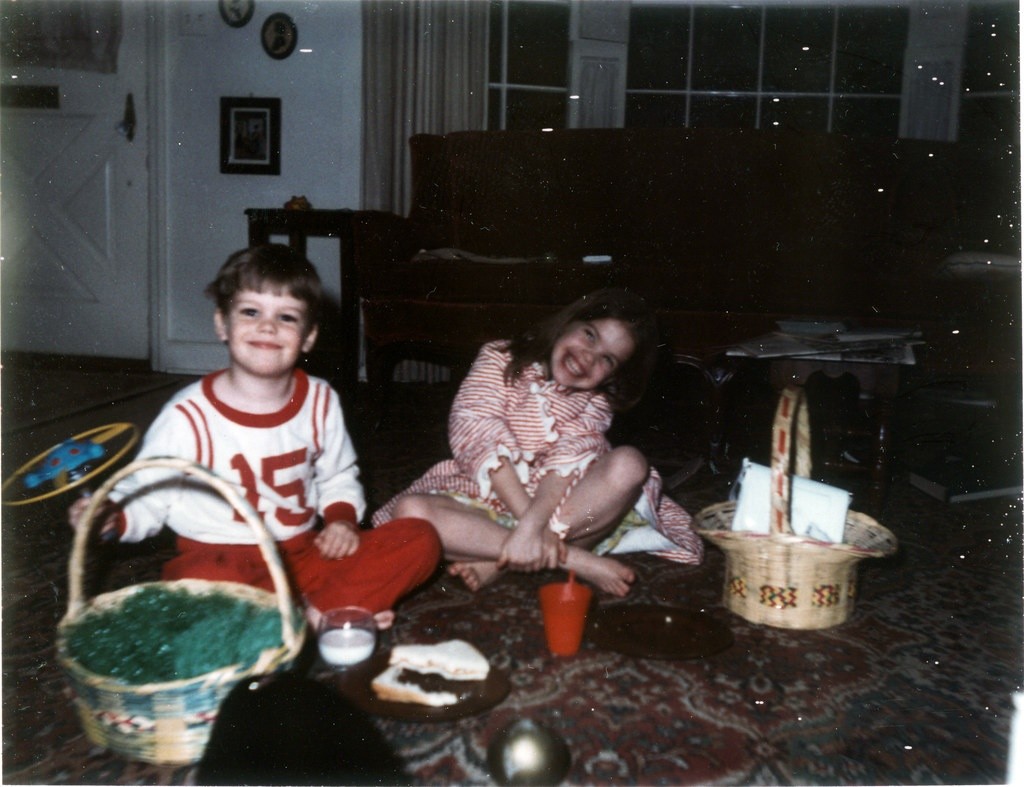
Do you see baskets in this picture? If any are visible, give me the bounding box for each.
[689,384,897,629]
[57,457,310,766]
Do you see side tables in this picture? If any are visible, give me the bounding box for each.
[242,208,393,406]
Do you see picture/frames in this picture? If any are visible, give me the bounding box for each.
[218,96,281,176]
[260,12,298,60]
[219,0,255,28]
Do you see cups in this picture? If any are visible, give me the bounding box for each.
[318,607,377,668]
[538,584,592,657]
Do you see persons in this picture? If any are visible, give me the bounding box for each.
[370,289,702,596]
[69,241,440,641]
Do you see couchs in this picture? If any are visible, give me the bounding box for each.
[364,122,1018,412]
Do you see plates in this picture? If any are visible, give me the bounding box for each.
[326,651,512,725]
[588,603,736,662]
